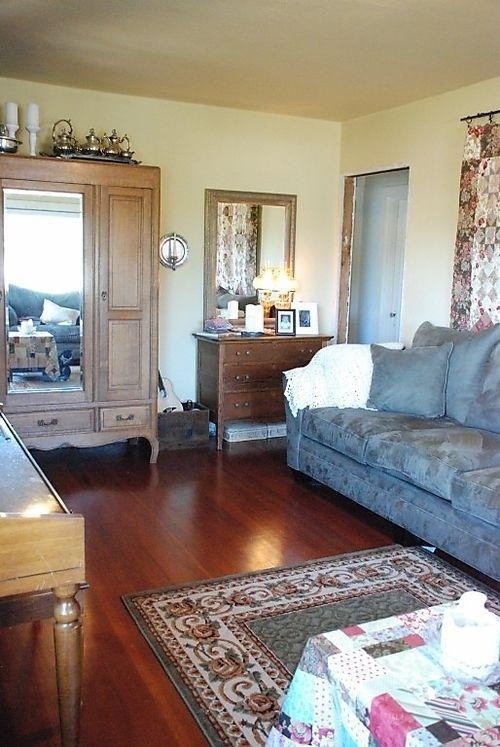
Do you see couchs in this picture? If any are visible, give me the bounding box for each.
[281,320,500,583]
[5,284,83,361]
[216,285,257,319]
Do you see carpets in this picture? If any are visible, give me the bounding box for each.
[121,540,500,745]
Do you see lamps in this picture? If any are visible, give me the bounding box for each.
[253,267,277,317]
[270,267,296,310]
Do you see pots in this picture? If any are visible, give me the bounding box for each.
[0,134,24,153]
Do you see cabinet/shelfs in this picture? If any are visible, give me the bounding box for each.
[1,155,162,464]
[191,332,334,450]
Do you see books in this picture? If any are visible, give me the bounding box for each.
[195,331,242,337]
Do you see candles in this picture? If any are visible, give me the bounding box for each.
[26,104,39,126]
[5,101,19,125]
[173,234,177,256]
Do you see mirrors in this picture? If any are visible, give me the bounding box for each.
[1,179,92,406]
[203,189,297,331]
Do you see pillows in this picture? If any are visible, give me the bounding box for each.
[365,341,455,420]
[461,341,500,433]
[40,298,80,326]
[411,321,500,425]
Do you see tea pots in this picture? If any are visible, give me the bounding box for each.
[82,127,101,157]
[51,118,78,154]
[103,127,132,158]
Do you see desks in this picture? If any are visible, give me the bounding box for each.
[1,408,89,745]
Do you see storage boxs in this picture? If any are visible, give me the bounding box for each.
[156,401,211,451]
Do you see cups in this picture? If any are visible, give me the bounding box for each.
[121,150,132,157]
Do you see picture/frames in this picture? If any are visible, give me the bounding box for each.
[294,302,319,335]
[275,308,297,337]
